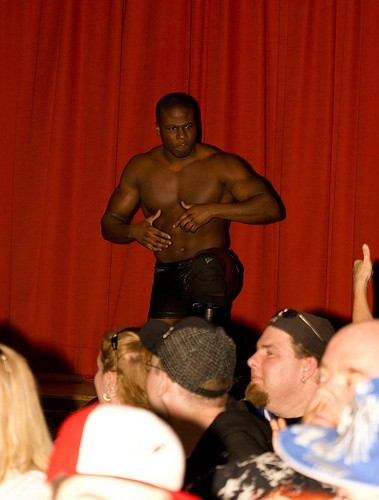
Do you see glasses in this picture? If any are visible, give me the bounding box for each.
[273,307,326,346]
[111,326,136,373]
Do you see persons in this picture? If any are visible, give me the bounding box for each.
[100,91,286,338]
[0,244,379,500]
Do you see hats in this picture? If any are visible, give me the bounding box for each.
[272,380,379,491]
[140,319,236,397]
[269,312,336,360]
[45,404,201,500]
